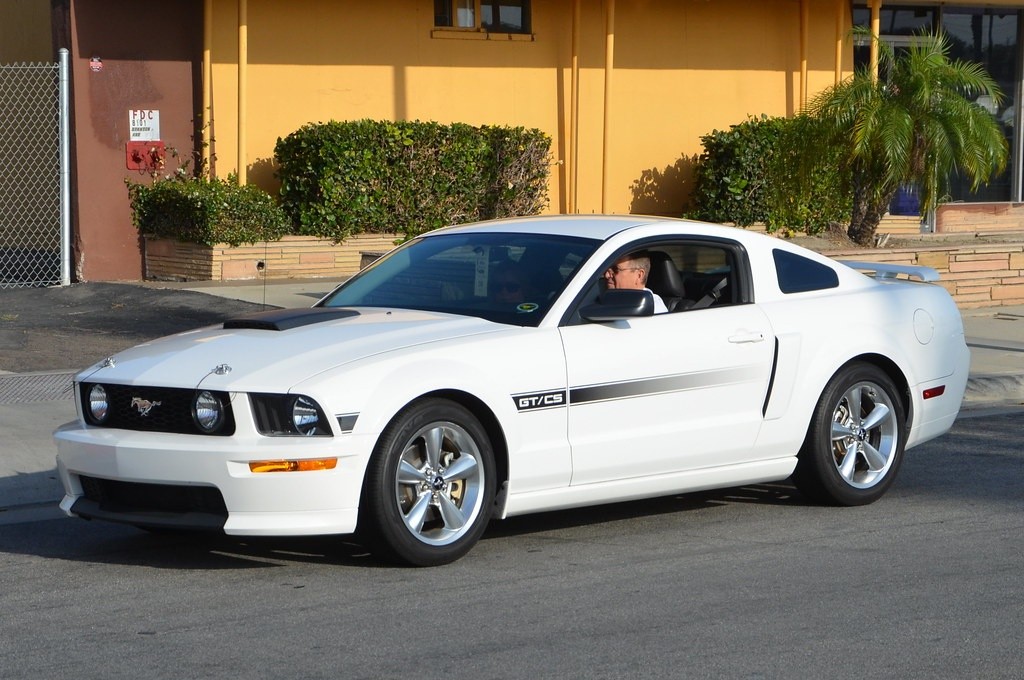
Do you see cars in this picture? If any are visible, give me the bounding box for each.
[52,215,971,566]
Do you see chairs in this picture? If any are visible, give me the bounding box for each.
[497,262,563,316]
[643,251,696,312]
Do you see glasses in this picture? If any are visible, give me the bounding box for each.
[609,264,640,276]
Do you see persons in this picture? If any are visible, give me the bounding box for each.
[605,251,669,314]
